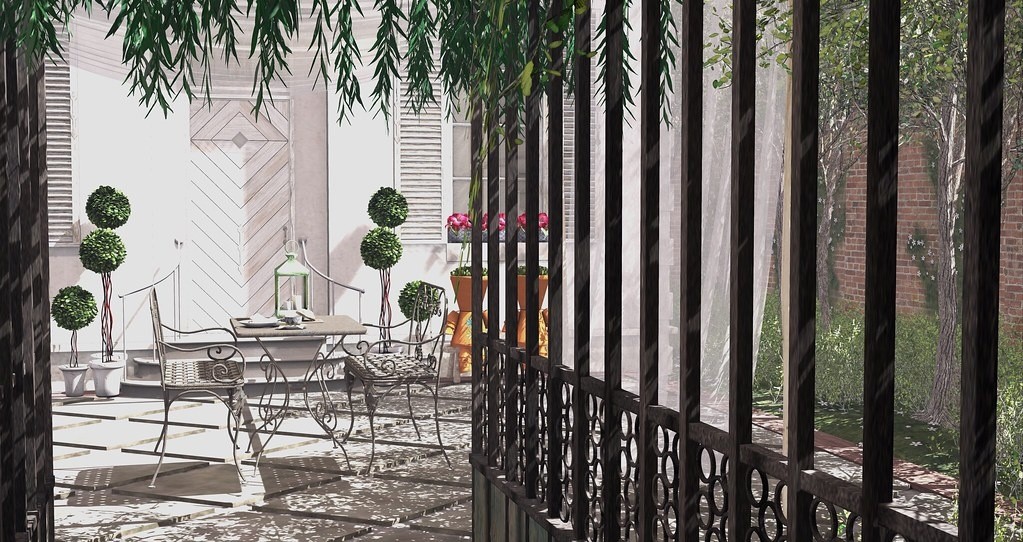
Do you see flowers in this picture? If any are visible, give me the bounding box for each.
[447,213,549,232]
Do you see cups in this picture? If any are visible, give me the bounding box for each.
[283,301,292,310]
[292,295,301,309]
[285,316,302,325]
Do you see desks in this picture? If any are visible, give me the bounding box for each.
[231,315,367,473]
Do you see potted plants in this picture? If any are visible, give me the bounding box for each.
[450,266,488,312]
[359,186,408,386]
[398,281,439,365]
[517,265,549,309]
[78,185,131,397]
[49,285,98,397]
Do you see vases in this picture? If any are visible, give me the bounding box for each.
[448,226,549,242]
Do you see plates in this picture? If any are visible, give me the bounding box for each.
[303,319,324,323]
[275,324,307,330]
[239,320,279,327]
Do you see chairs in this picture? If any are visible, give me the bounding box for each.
[341,281,451,476]
[148,286,246,488]
